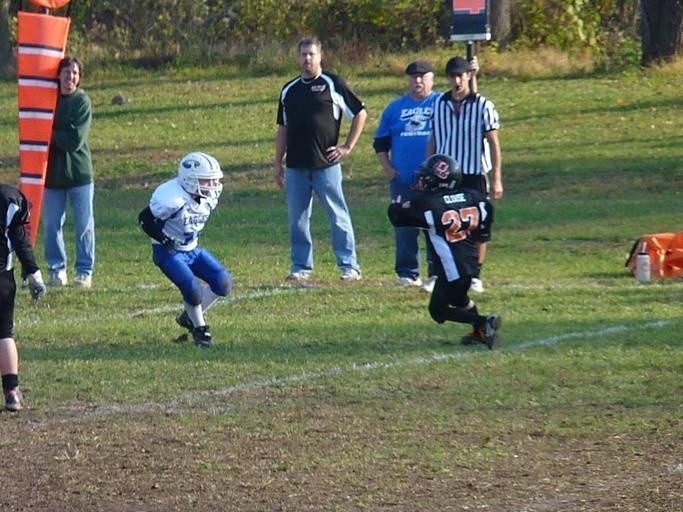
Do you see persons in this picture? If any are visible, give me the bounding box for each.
[0,181,46,414]
[386,154,504,349]
[417,58,505,294]
[269,35,368,279]
[371,56,481,290]
[138,151,232,347]
[40,56,97,290]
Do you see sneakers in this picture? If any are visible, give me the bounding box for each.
[418,274,438,293]
[339,268,363,281]
[457,310,506,352]
[71,270,92,289]
[468,277,485,294]
[283,272,310,283]
[3,384,26,411]
[47,270,68,287]
[399,275,422,288]
[173,308,213,347]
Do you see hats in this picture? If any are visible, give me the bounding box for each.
[443,56,471,76]
[403,60,433,76]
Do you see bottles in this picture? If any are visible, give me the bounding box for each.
[635,252,651,284]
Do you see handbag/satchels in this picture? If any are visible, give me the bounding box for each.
[623,231,683,280]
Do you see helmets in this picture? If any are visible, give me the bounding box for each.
[174,151,224,196]
[418,152,463,194]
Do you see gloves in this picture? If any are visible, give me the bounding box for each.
[23,268,48,302]
